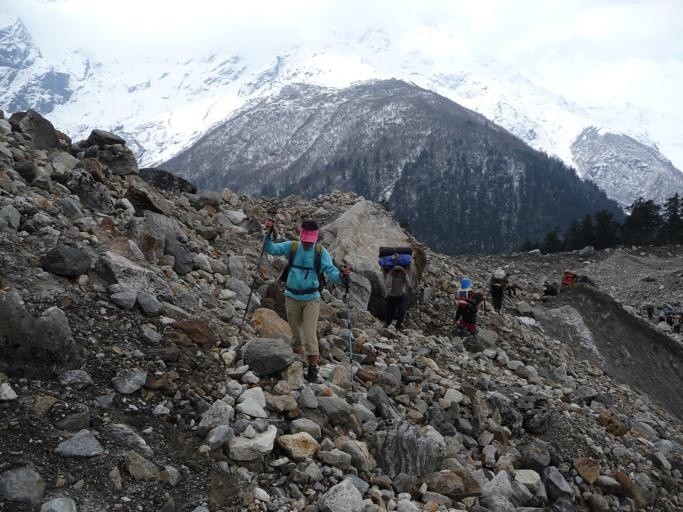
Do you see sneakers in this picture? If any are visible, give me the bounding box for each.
[307,364,318,383]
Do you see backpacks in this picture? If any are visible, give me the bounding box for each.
[279,240,327,291]
[378,247,413,269]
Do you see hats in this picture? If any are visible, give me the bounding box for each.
[300,225,319,243]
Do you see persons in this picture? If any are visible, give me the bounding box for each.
[453,290,485,341]
[488,265,511,313]
[259,216,350,385]
[453,276,485,325]
[380,265,413,332]
[541,269,578,296]
[645,301,683,335]
